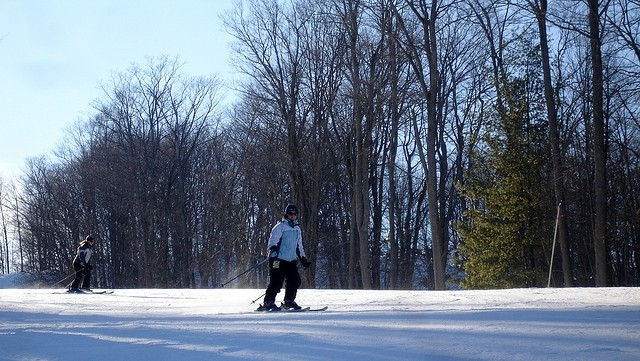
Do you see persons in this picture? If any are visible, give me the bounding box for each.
[263,204,311,311]
[71,236,95,293]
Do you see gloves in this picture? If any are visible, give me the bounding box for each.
[270,246,278,258]
[85,264,92,268]
[301,258,309,268]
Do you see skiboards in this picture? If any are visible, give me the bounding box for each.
[218,306,328,315]
[51,291,114,294]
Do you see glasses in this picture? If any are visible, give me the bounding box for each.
[289,212,297,216]
[89,240,93,242]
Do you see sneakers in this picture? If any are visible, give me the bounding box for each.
[69,287,82,293]
[264,302,281,310]
[281,301,301,309]
[82,287,92,292]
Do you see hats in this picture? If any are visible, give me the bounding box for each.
[285,204,298,213]
[86,235,93,242]
